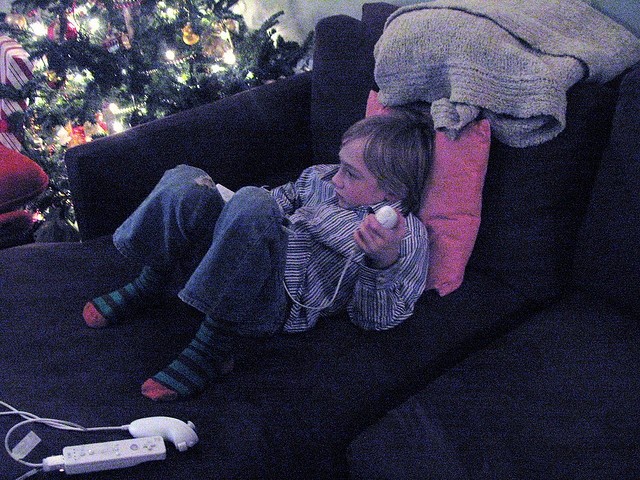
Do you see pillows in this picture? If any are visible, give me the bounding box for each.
[363,91,491,297]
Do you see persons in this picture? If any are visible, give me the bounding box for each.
[83,114,435,402]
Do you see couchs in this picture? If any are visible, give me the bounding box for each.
[1,0,637,480]
